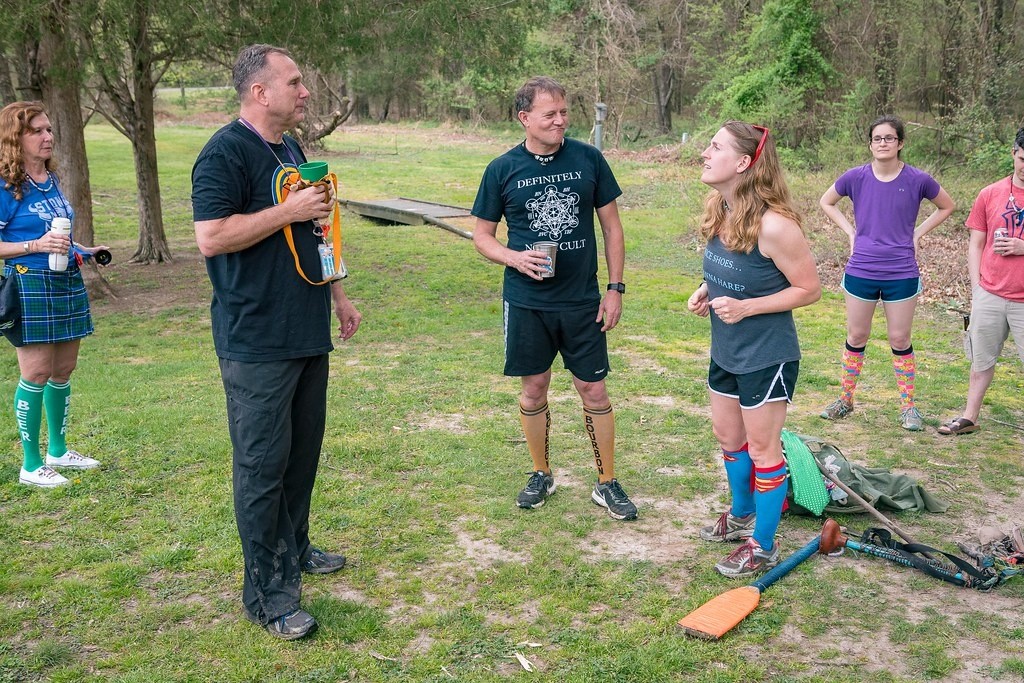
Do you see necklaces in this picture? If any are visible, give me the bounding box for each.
[1009,173,1024,213]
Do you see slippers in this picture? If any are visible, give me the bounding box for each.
[939,418,979,433]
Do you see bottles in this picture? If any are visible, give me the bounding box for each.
[48,217,72,271]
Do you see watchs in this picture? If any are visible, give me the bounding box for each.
[606,282,626,293]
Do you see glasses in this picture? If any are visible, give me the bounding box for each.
[871,135,898,143]
[750,126,769,169]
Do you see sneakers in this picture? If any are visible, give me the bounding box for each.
[714,537,779,578]
[699,508,757,542]
[821,401,855,420]
[19,465,69,488]
[900,407,922,431]
[299,544,346,573]
[246,606,317,640]
[46,451,100,469]
[518,469,555,509]
[591,478,638,521]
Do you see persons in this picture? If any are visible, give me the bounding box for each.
[938,126,1024,434]
[191,45,361,641]
[469,76,637,520]
[687,122,821,578]
[0,102,109,488]
[819,116,955,431]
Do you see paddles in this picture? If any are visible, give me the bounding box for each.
[674,525,848,642]
[817,460,940,564]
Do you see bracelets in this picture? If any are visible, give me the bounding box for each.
[24,240,39,254]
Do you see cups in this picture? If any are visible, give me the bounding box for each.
[297,161,328,184]
[532,241,558,277]
